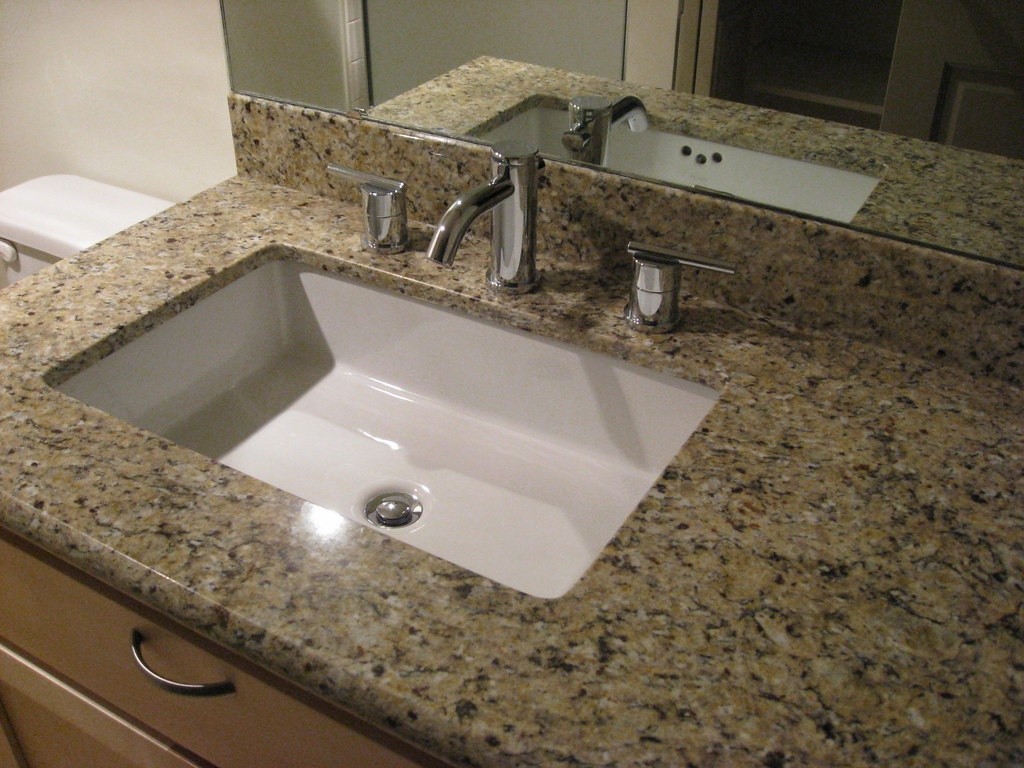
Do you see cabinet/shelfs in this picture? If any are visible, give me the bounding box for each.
[1,527,449,767]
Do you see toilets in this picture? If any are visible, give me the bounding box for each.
[0,163,178,282]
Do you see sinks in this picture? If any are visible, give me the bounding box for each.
[33,231,742,603]
[463,84,890,226]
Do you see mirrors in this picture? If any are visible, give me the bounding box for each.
[220,0,1024,269]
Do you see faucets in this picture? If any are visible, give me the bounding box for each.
[561,86,652,165]
[426,139,546,296]
